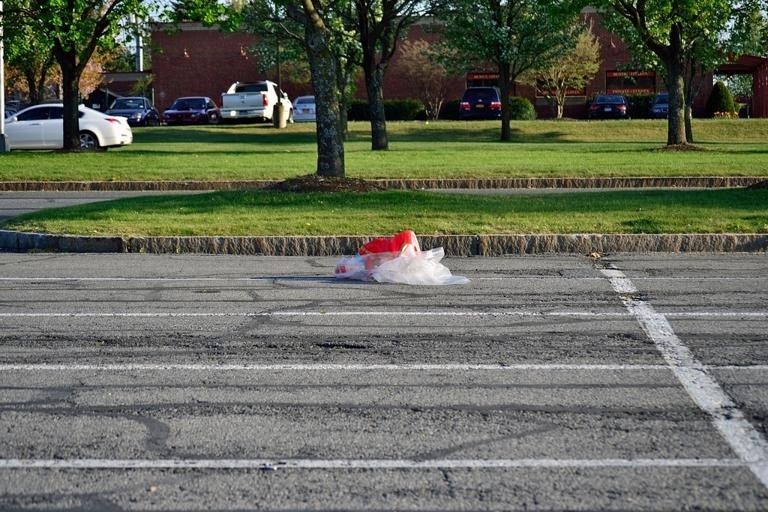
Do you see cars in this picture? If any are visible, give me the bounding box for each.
[650,92,669,117]
[3,103,133,150]
[459,86,502,119]
[293,95,316,121]
[589,94,630,119]
[162,97,220,125]
[105,97,160,126]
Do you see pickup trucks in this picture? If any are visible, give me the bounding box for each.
[220,79,294,123]
